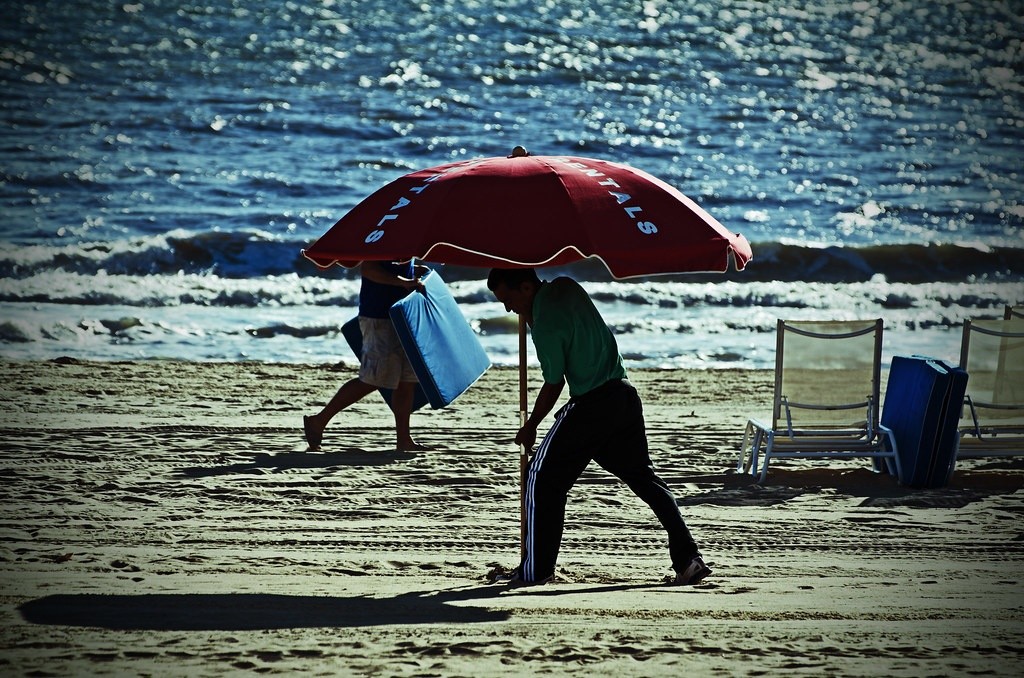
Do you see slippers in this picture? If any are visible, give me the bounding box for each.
[397,443,435,453]
[303,416,323,450]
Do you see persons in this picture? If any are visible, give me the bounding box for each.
[303,260,433,451]
[488,268,713,585]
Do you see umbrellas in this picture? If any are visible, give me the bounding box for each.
[300,146,753,564]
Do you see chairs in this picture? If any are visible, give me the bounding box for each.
[738,318,903,485]
[946,304,1024,484]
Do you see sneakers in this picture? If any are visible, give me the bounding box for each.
[675,554,712,587]
